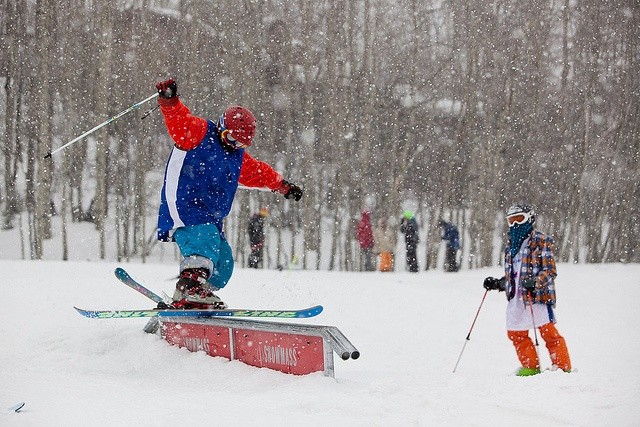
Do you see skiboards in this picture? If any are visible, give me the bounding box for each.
[72,268,323,319]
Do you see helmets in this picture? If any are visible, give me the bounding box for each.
[504,202,538,227]
[215,104,258,147]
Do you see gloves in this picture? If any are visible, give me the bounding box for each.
[155,78,179,108]
[483,276,499,291]
[270,179,304,202]
[523,279,536,291]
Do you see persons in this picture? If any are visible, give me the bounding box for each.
[483,204,573,377]
[357,210,376,271]
[438,219,459,272]
[246,207,270,268]
[374,218,395,271]
[148,76,303,308]
[399,212,422,271]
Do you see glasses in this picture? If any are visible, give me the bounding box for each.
[219,116,247,150]
[505,207,537,228]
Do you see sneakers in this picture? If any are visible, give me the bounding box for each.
[172,278,185,302]
[181,266,225,306]
[515,366,542,377]
